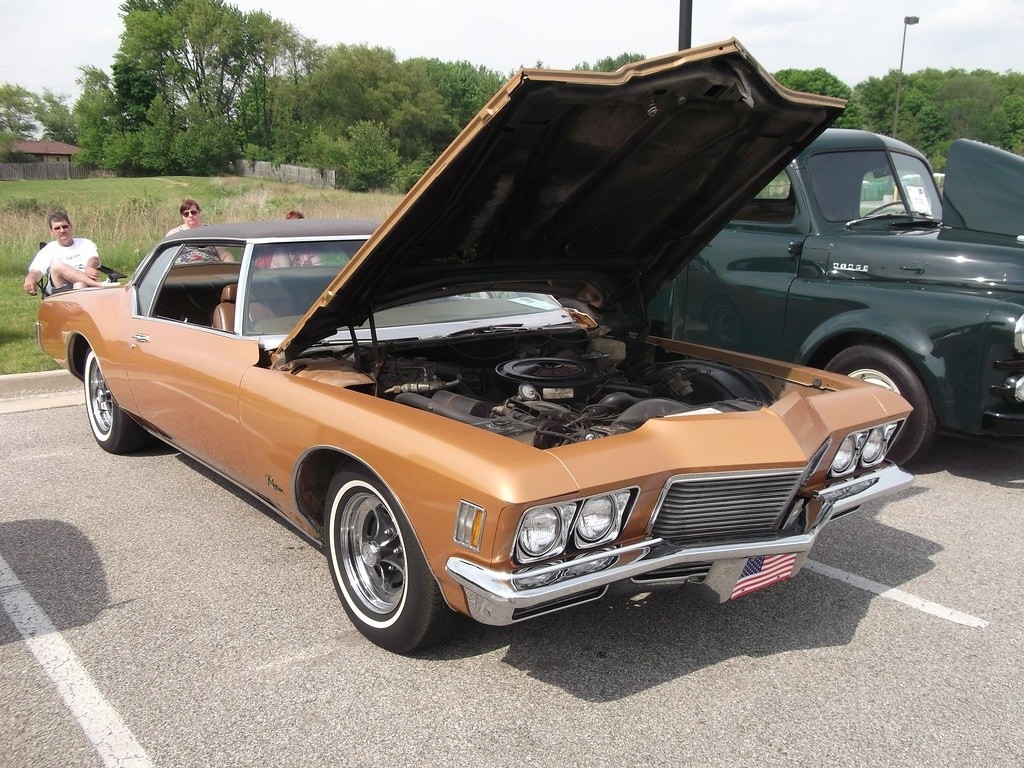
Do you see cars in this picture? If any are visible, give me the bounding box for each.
[32,42,916,660]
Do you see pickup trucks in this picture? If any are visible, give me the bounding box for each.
[649,126,1023,470]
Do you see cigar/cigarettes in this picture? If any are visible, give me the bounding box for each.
[27,291,37,293]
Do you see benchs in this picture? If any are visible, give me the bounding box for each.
[152,274,339,324]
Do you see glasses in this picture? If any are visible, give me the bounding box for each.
[182,210,199,218]
[52,225,70,231]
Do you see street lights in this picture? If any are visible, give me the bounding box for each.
[892,14,921,138]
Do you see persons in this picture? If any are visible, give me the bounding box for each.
[23,211,121,299]
[163,198,238,265]
[252,209,324,271]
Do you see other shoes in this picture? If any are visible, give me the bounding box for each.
[101,282,122,287]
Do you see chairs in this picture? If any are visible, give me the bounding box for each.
[213,282,292,331]
[35,240,128,300]
